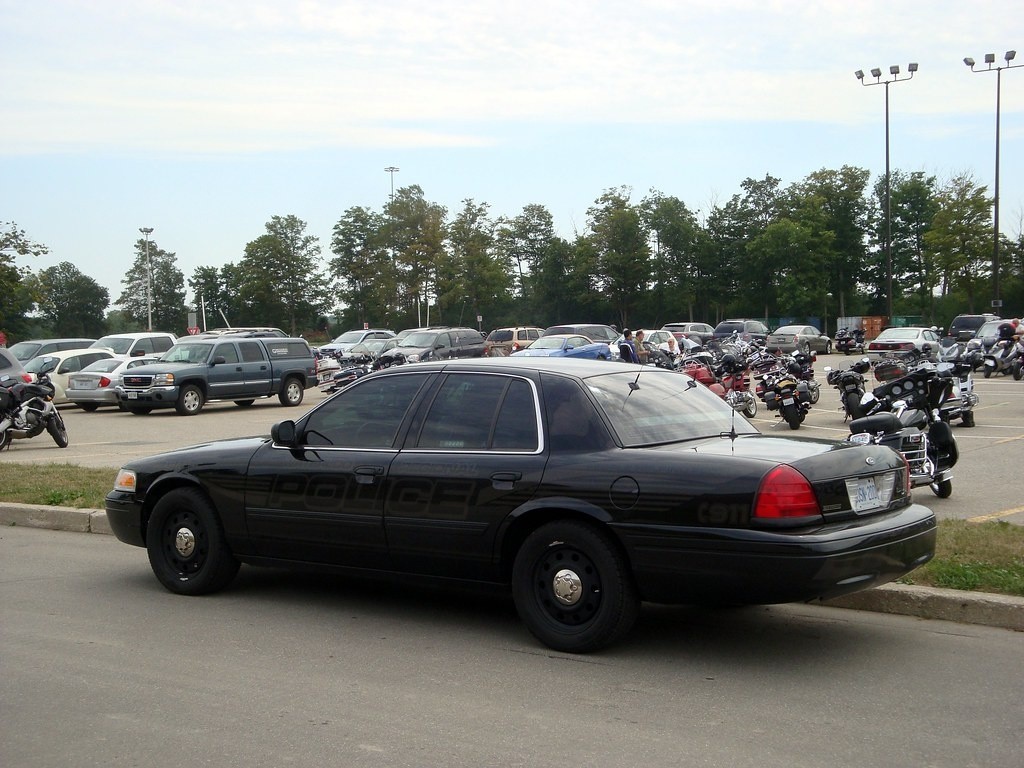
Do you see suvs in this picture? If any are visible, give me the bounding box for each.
[712,319,769,352]
[949,314,999,344]
[661,323,715,349]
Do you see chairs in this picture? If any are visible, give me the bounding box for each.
[418,422,486,449]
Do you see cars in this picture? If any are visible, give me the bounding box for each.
[767,326,834,355]
[610,328,679,363]
[868,326,942,366]
[0,298,545,415]
[105,361,940,651]
[543,323,623,347]
[976,318,1020,352]
[510,336,611,362]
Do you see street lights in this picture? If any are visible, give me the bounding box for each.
[386,167,400,264]
[962,50,1024,314]
[140,226,154,330]
[855,64,918,326]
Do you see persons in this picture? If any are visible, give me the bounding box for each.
[663,338,679,354]
[618,330,640,363]
[999,318,1021,342]
[632,329,651,363]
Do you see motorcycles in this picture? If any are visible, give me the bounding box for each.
[659,331,1024,498]
[835,326,867,355]
[0,358,71,453]
